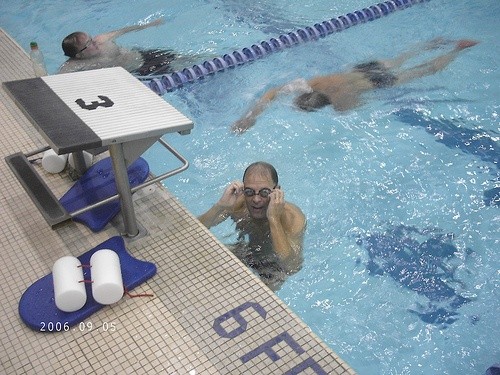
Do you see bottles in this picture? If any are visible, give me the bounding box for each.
[28,41,48,78]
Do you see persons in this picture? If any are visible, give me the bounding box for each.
[57,18,177,81]
[196,162,305,262]
[232,36,481,134]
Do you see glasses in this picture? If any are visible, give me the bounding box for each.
[78,33,93,53]
[243,185,277,198]
